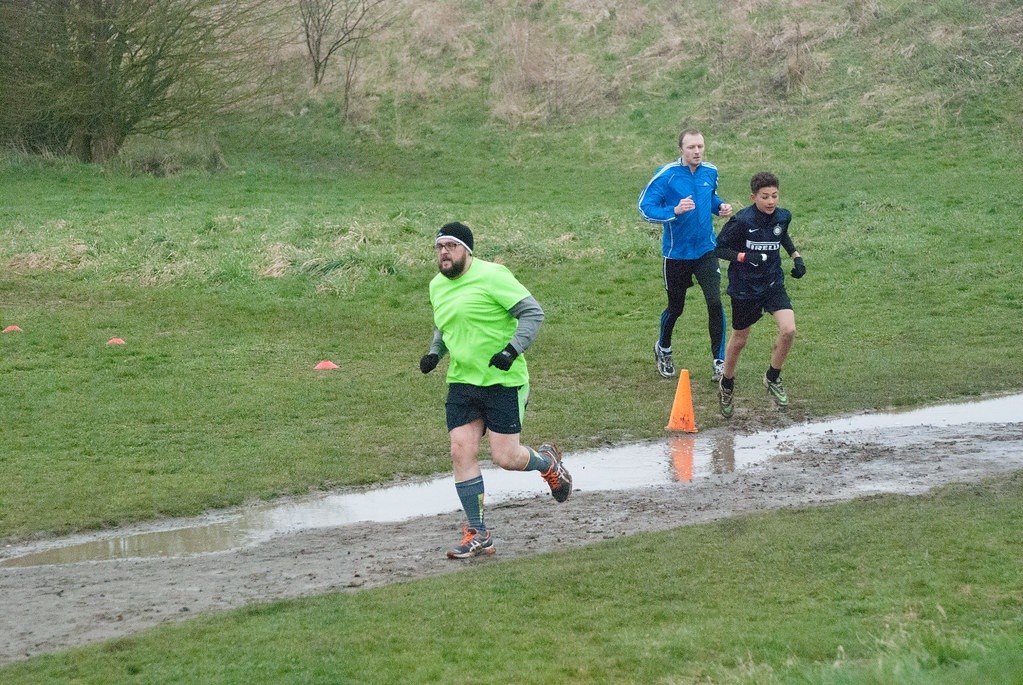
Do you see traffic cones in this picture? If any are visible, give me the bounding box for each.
[665,369,699,433]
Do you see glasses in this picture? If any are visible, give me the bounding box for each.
[436,241,464,254]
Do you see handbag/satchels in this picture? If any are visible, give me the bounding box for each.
[791,256,807,279]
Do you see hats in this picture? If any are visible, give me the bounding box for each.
[434,222,475,255]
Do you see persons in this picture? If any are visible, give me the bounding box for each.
[638,129,732,383]
[420,222,572,558]
[714,171,806,418]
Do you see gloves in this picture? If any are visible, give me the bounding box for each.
[420,352,439,374]
[737,252,770,271]
[489,344,520,371]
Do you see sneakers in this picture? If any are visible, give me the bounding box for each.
[539,440,572,505]
[718,374,736,418]
[710,359,725,382]
[763,371,789,407]
[653,341,676,379]
[445,525,496,561]
